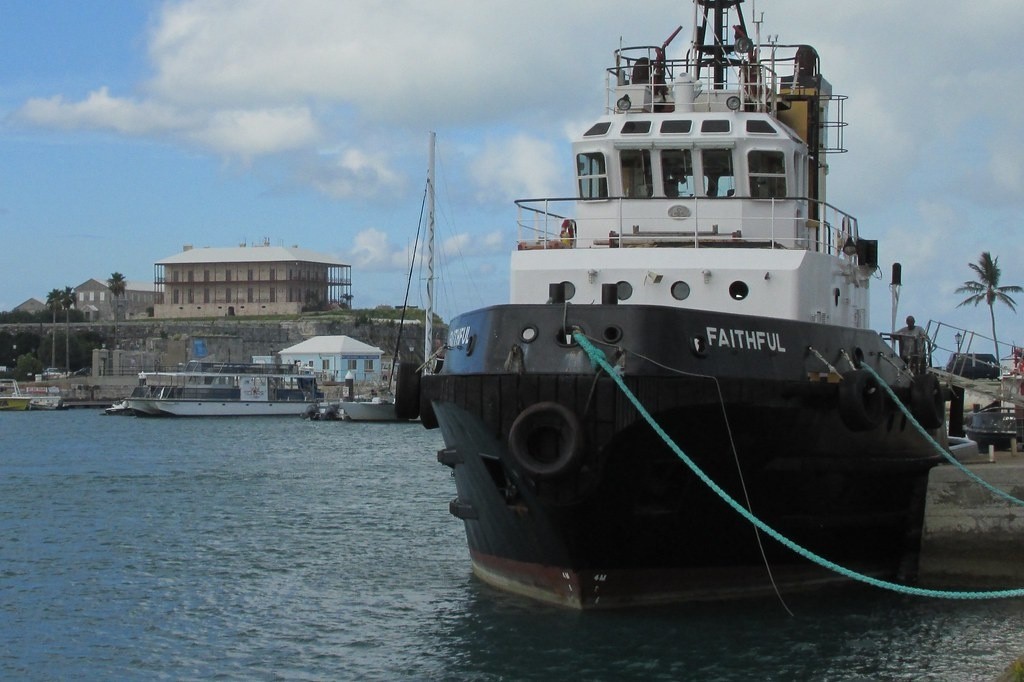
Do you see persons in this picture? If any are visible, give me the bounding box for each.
[891,315,930,375]
[288,358,310,374]
[138,370,147,386]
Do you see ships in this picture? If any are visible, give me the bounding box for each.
[419,0,952,618]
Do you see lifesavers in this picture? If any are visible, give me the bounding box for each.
[395,362,422,420]
[419,394,440,430]
[560,219,574,244]
[837,368,887,432]
[910,373,945,428]
[508,402,584,481]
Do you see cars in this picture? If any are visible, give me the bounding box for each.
[42,368,61,379]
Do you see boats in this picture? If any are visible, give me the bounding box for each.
[339,397,410,422]
[104,401,136,416]
[0,378,33,411]
[127,359,341,418]
